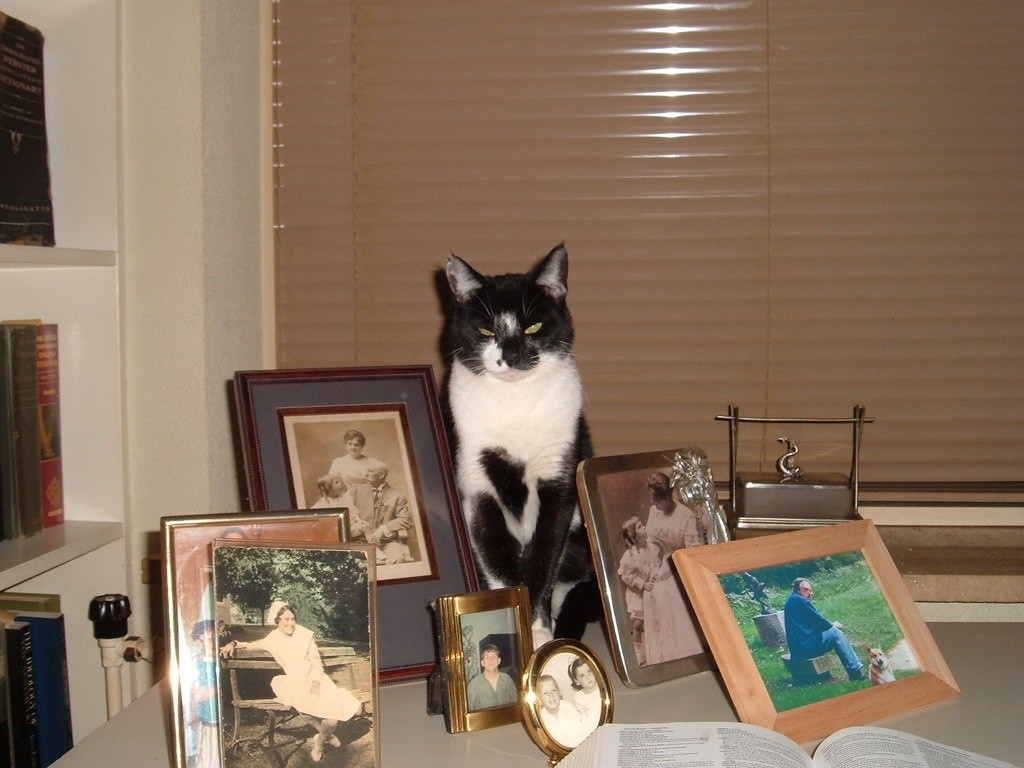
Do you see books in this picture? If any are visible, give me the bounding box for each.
[0,318,65,542]
[0,592,75,768]
[0,11,56,247]
[554,722,1018,768]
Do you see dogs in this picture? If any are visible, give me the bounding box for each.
[868,644,896,686]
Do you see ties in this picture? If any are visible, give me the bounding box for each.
[374,491,378,504]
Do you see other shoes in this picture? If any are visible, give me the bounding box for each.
[328,735,341,748]
[311,734,325,762]
[850,671,868,680]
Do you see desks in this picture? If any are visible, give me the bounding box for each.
[47,597,1024,768]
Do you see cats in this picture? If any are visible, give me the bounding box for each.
[442,243,602,648]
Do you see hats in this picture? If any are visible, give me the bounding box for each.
[267,602,288,623]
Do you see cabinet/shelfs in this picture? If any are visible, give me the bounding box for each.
[0,217,128,592]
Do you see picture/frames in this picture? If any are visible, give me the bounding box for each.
[233,364,484,687]
[578,448,730,689]
[161,508,378,768]
[673,519,961,745]
[438,585,534,734]
[521,640,613,767]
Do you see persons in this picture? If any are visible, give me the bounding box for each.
[310,429,413,565]
[784,577,869,681]
[536,658,601,738]
[190,527,251,768]
[467,644,518,711]
[618,473,706,667]
[219,602,361,762]
